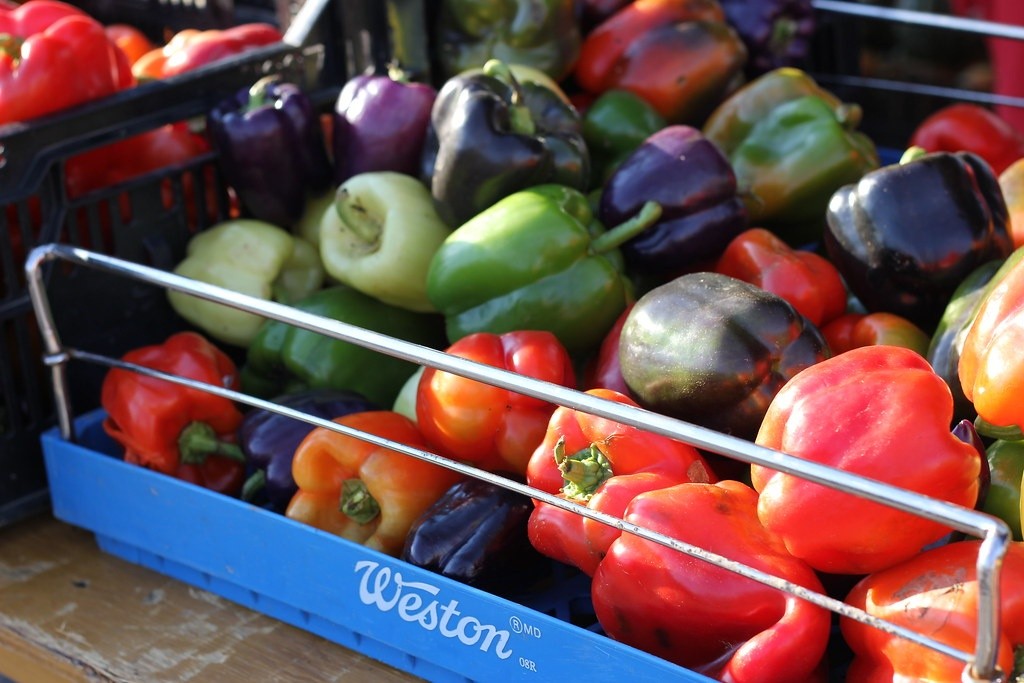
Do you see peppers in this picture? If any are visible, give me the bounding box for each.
[0,0,1024,683]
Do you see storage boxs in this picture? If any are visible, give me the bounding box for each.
[0,0,394,533]
